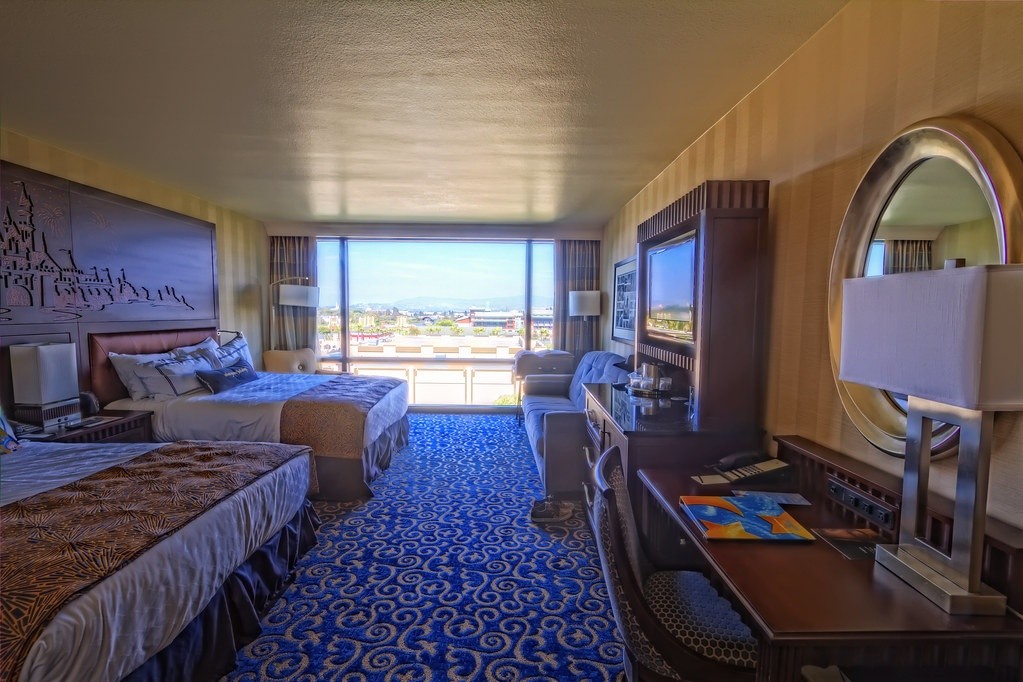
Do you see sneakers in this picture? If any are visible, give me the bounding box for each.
[531,501,573,522]
[533,494,574,511]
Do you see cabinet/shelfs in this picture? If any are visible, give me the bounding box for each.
[582,176,771,569]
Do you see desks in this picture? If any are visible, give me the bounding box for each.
[639,463,1023,682]
[516,374,523,425]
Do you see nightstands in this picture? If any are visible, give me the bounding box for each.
[46,408,153,444]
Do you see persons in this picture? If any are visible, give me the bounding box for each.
[819,528,882,540]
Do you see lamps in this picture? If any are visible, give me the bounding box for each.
[568,291,602,355]
[268,277,321,349]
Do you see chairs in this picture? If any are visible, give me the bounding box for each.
[824,113,1023,463]
[262,348,317,374]
[590,446,758,682]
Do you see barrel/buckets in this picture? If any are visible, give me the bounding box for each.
[642,360,668,389]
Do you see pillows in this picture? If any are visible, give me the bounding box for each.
[194,357,258,395]
[524,380,568,395]
[197,331,255,371]
[0,405,22,454]
[133,353,215,400]
[109,351,175,401]
[173,336,220,362]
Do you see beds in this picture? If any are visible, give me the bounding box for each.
[87,332,411,500]
[0,440,321,682]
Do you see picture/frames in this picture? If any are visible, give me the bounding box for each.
[611,255,637,345]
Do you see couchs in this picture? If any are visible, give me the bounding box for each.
[521,348,636,500]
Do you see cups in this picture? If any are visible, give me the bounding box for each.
[630,395,642,406]
[627,373,642,389]
[640,406,652,415]
[641,376,655,388]
[689,386,695,405]
[641,398,653,407]
[659,399,671,408]
[658,376,673,390]
[688,405,695,421]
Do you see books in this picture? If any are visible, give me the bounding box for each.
[679,495,817,542]
[810,527,893,560]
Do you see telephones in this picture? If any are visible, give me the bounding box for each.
[713,447,794,485]
